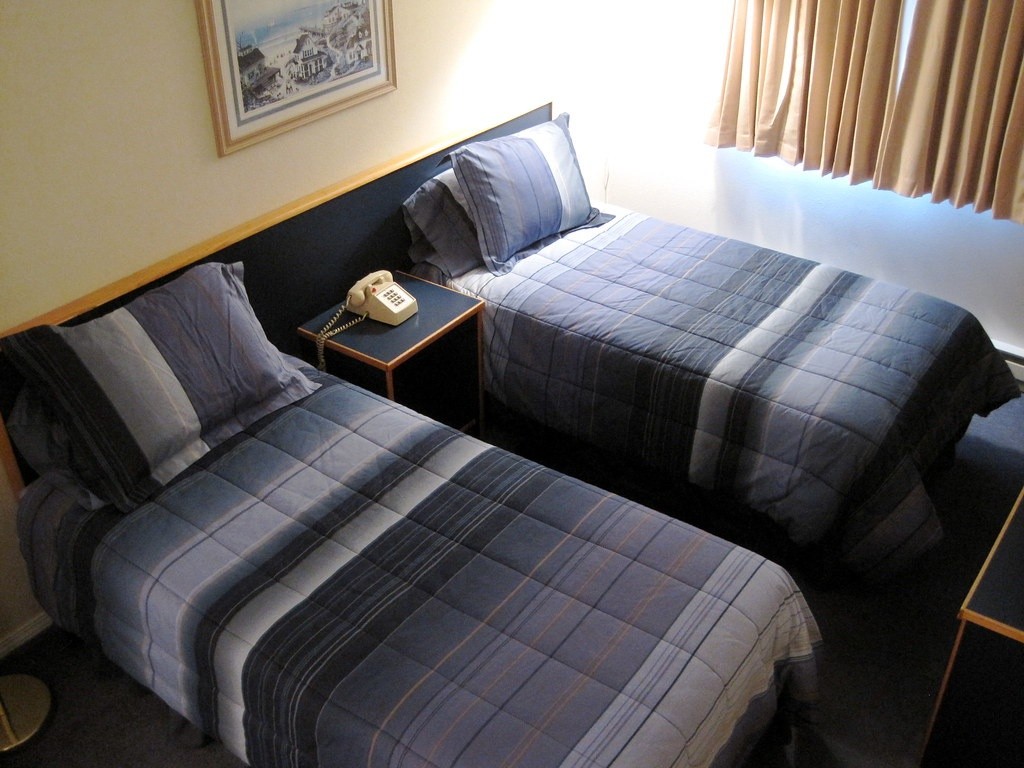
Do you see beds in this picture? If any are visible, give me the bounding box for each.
[0,347,820,768]
[411,211,1024,577]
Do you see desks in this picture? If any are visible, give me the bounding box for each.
[921,484,1024,768]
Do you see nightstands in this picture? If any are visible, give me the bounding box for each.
[300,270,487,438]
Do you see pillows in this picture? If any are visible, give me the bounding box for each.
[436,112,615,279]
[400,168,486,279]
[0,260,321,510]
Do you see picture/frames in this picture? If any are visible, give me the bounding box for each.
[191,0,399,159]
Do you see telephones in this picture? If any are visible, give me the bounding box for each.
[345,270,419,327]
